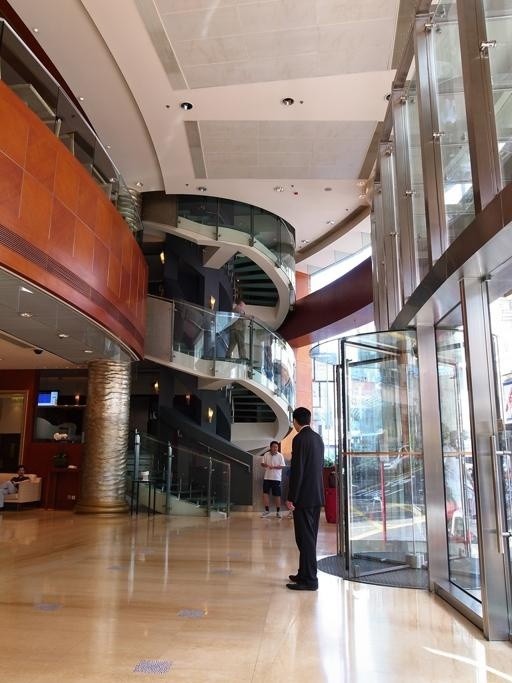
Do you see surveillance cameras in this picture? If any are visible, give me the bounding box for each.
[34,348,43,353]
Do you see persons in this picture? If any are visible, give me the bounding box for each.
[0,466,29,511]
[442,430,478,544]
[285,408,325,590]
[225,299,247,359]
[260,441,286,518]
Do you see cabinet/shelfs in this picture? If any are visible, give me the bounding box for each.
[21,372,88,509]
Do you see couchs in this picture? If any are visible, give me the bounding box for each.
[0,472,42,511]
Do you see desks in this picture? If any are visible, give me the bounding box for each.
[38,467,84,511]
[131,480,156,516]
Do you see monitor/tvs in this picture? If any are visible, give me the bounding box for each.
[36,390,59,406]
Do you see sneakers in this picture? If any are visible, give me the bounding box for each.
[277,512,281,518]
[260,510,270,517]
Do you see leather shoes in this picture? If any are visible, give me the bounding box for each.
[286,575,317,590]
